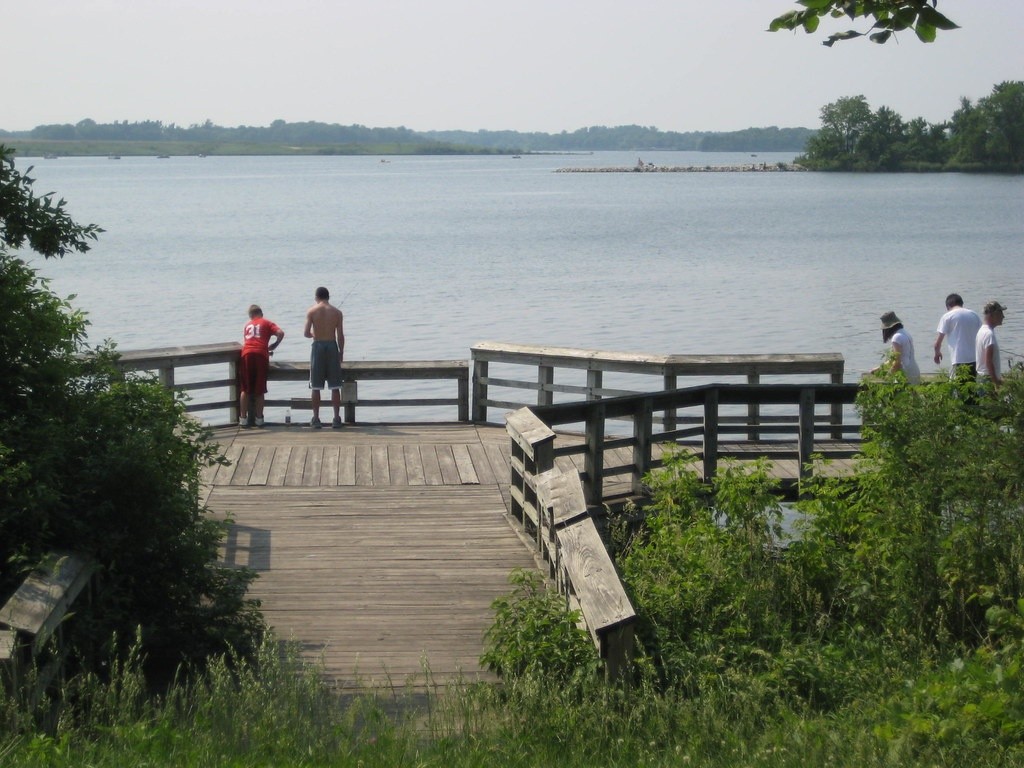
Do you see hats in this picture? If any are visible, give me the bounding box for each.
[983,300,1007,313]
[880,311,901,329]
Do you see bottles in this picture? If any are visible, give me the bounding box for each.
[285,409,291,427]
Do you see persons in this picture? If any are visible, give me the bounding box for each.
[239,305,284,427]
[976,301,1007,398]
[934,293,983,429]
[304,287,345,428]
[870,311,921,385]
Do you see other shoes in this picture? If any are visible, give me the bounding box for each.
[255,415,264,425]
[310,417,322,428]
[239,416,248,426]
[332,416,342,428]
